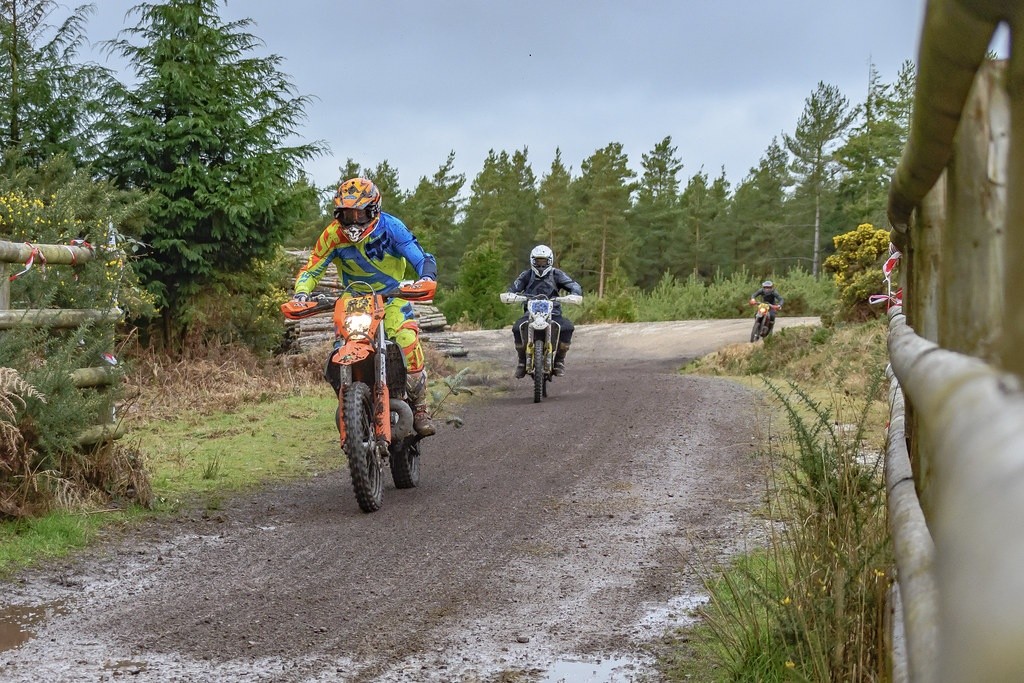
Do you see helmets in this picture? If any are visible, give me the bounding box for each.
[762,281,774,295]
[530,245,553,278]
[333,178,382,243]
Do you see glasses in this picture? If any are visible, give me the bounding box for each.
[333,209,378,226]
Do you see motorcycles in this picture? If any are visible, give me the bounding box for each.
[279,278,436,511]
[499,292,585,403]
[749,299,782,344]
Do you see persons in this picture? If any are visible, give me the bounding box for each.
[750,281,784,337]
[287,178,437,435]
[505,245,582,379]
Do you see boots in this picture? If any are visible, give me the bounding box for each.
[553,341,571,377]
[404,365,437,437]
[514,346,527,379]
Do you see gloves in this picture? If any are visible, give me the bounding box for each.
[776,304,782,310]
[292,292,311,302]
[750,299,757,306]
